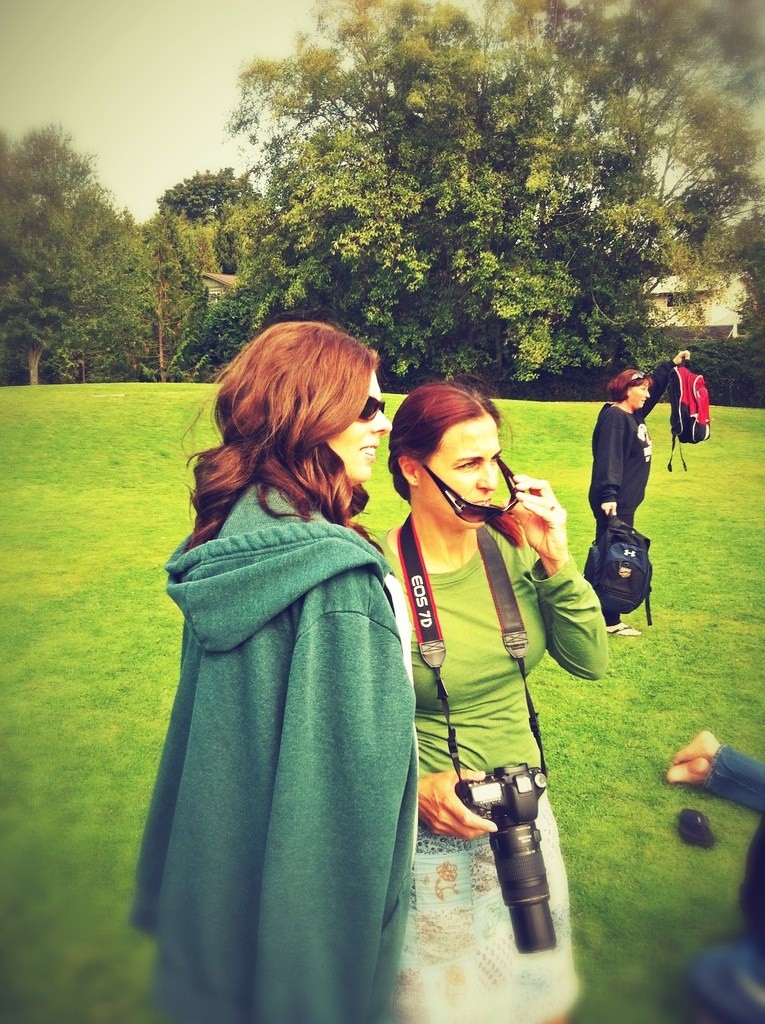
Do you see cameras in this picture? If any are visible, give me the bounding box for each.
[455,763,557,954]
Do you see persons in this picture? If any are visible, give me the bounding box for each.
[123,317,420,1023]
[588,347,692,636]
[663,727,765,1024]
[373,379,609,1024]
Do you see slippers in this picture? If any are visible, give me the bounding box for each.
[607,626,642,637]
[679,809,715,847]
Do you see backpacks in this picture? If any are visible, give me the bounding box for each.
[668,356,711,444]
[589,509,652,614]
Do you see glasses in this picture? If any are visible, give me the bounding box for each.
[632,371,646,380]
[422,459,525,523]
[358,396,386,421]
